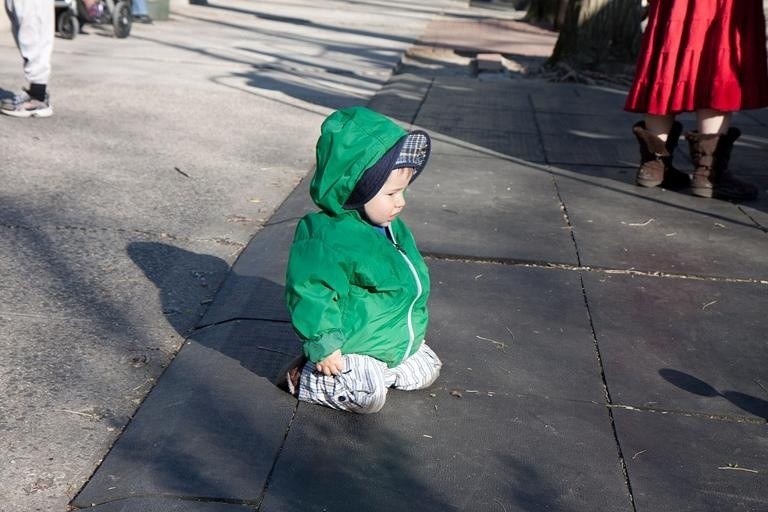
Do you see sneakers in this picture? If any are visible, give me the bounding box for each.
[0,86,54,119]
[276,353,307,391]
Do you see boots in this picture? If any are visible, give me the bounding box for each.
[681,124,760,203]
[631,117,690,190]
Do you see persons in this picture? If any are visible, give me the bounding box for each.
[622,0,768,202]
[0,0,56,118]
[275,105,444,414]
[130,0,152,23]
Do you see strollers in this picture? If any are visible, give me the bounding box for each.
[53,1,155,44]
